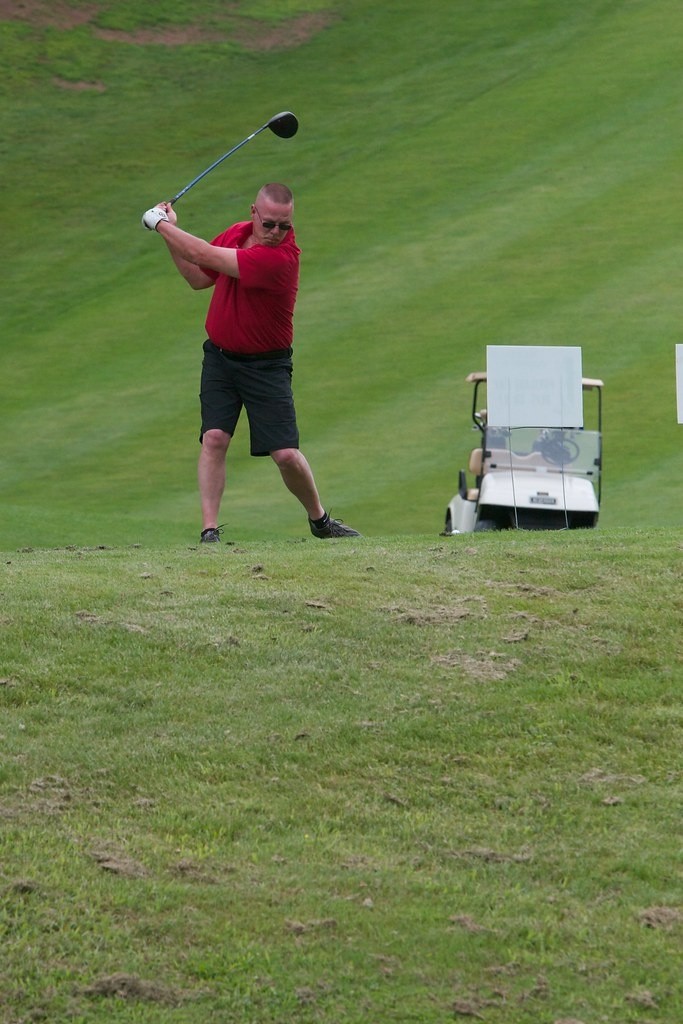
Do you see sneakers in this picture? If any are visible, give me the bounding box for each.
[308,516,361,539]
[198,523,228,544]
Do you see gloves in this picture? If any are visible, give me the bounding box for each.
[140,206,170,232]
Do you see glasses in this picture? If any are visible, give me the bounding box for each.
[255,207,292,231]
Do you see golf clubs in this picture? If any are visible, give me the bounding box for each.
[146,109,301,228]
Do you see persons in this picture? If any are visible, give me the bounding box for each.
[142,182,358,544]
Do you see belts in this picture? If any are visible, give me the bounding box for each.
[212,343,292,362]
